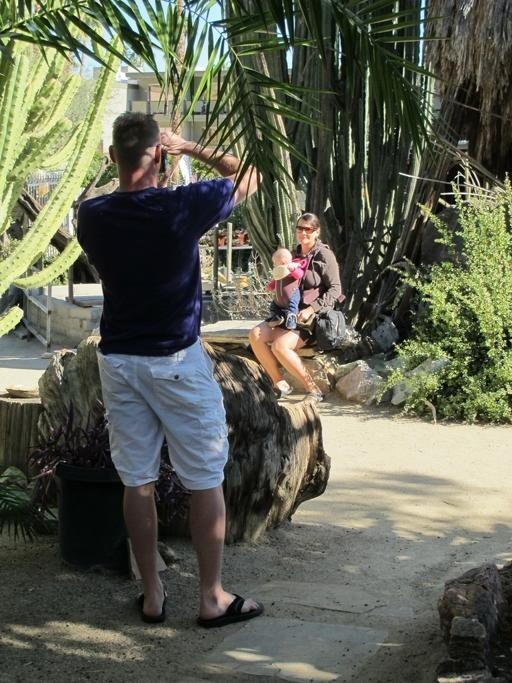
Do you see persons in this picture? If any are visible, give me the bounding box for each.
[261,247,307,329]
[76,110,266,625]
[249,212,341,403]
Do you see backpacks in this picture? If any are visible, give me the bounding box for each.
[314,302,347,351]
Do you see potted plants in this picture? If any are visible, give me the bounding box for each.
[25,401,143,567]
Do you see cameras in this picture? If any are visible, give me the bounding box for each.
[160,149,167,173]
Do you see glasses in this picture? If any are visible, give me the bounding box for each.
[295,225,316,235]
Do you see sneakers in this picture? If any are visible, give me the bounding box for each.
[266,314,297,330]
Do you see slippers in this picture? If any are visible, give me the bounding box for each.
[273,386,326,405]
[135,587,170,623]
[196,591,265,628]
